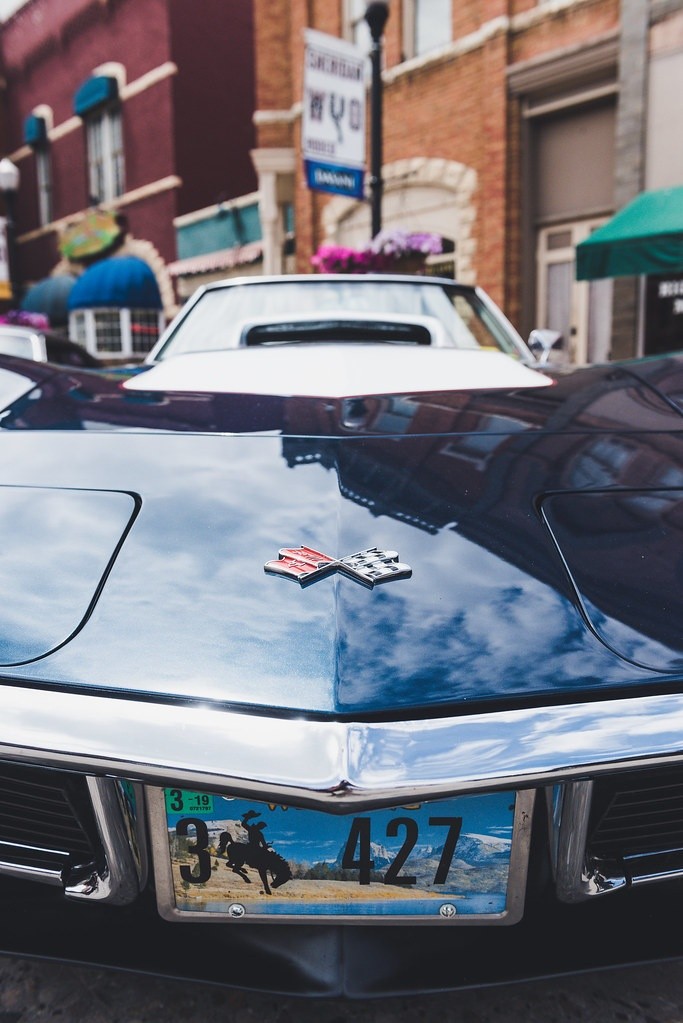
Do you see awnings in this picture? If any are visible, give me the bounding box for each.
[575,186,683,281]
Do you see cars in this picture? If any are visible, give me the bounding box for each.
[0,324,102,407]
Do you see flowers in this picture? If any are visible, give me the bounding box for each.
[0,310,52,332]
[309,245,373,273]
[366,220,443,260]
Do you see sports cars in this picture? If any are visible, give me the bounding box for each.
[1,269,683,997]
